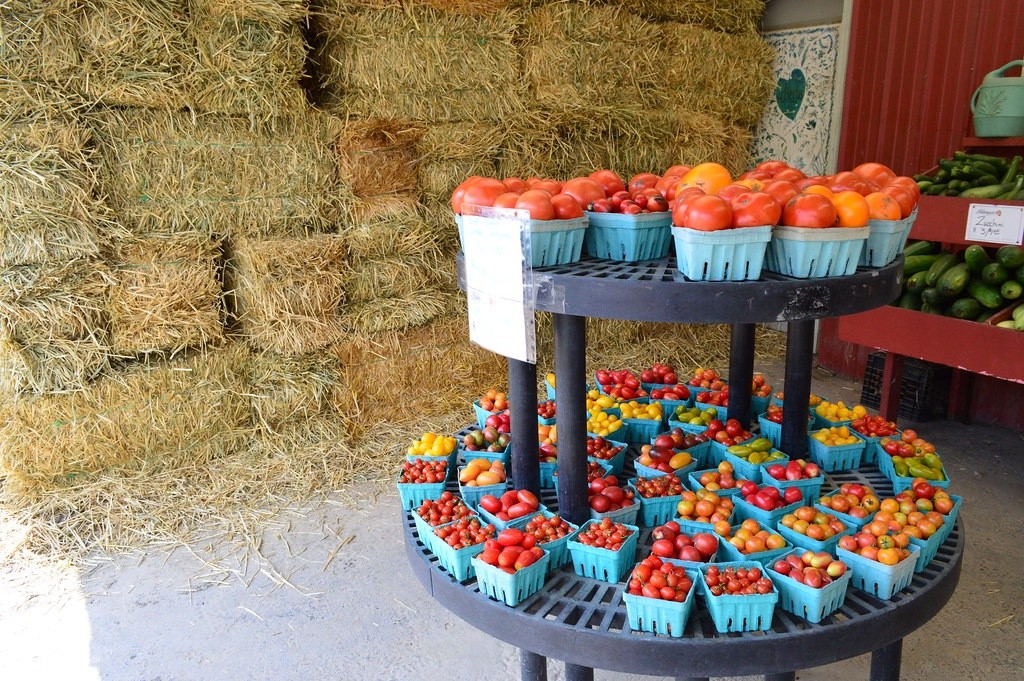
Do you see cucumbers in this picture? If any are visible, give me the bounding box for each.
[889,151,1023,331]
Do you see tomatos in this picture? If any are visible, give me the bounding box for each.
[398,156,956,634]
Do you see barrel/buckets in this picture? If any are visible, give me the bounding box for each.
[970,60,1024,138]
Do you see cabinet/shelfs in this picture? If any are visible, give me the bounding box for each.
[837,135,1024,425]
[397,250,966,681]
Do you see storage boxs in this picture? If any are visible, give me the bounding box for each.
[392,157,962,636]
